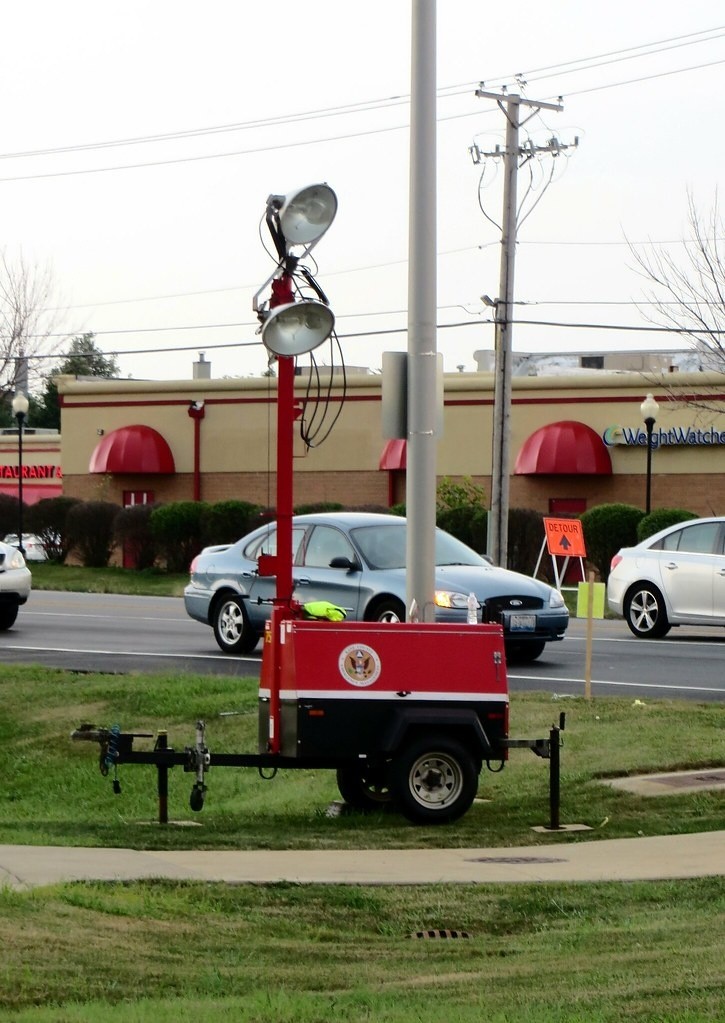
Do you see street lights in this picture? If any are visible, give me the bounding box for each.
[640,392,660,515]
[12,390,29,553]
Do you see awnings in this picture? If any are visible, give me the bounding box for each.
[90,425,177,474]
[378,439,407,470]
[514,420,613,475]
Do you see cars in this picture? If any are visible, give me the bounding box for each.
[606,514,725,640]
[182,512,569,663]
[2,527,63,564]
[0,541,32,631]
